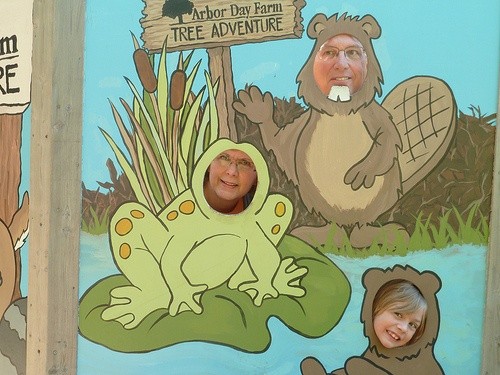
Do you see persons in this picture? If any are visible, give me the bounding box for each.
[314,34,367,96]
[372,280,428,349]
[203,149,258,214]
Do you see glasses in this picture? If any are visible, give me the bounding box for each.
[215,151,256,171]
[317,45,366,62]
